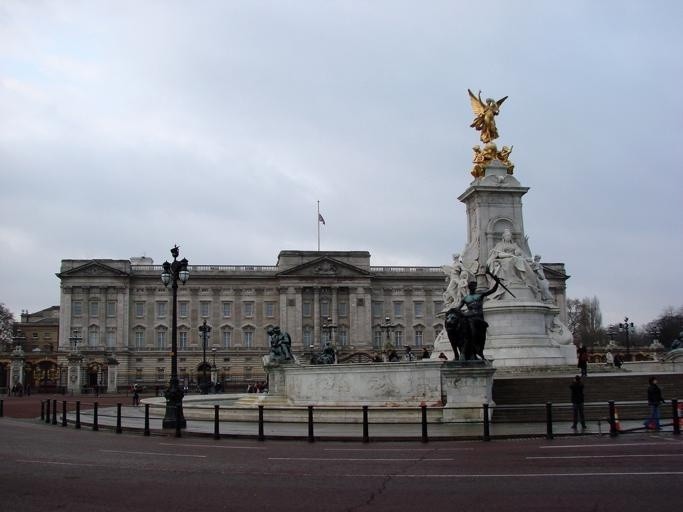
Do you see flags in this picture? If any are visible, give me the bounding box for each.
[318,213,324,224]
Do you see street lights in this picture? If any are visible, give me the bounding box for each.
[322,316,337,343]
[648,325,661,343]
[197,318,212,362]
[159,243,191,429]
[8,328,25,395]
[379,315,395,341]
[618,315,635,355]
[210,344,218,384]
[65,327,83,394]
[606,324,617,344]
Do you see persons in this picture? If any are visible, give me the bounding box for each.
[442,228,553,307]
[477,90,500,142]
[569,375,586,429]
[575,345,588,377]
[267,327,291,360]
[187,377,269,395]
[128,384,143,405]
[371,347,447,362]
[471,143,514,179]
[643,379,664,430]
[455,276,499,360]
[321,343,334,361]
[605,350,613,366]
[9,381,33,397]
[613,353,623,370]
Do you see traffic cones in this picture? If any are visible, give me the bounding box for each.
[614,407,621,430]
